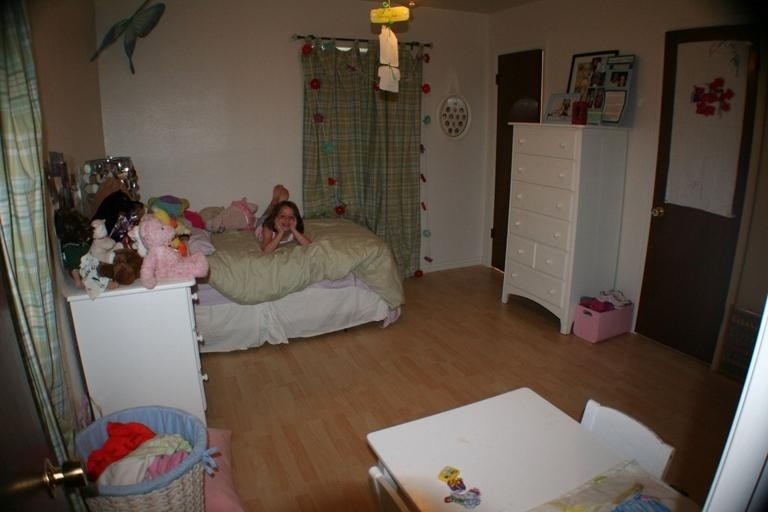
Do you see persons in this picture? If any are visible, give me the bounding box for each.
[252,183,311,253]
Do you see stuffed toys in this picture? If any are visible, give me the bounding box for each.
[55,177,259,301]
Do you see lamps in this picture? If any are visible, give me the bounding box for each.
[369,0,412,25]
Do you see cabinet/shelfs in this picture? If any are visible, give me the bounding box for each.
[67,265,209,430]
[502,120,632,335]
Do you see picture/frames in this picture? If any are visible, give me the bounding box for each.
[435,91,473,142]
[564,50,620,116]
[541,91,583,124]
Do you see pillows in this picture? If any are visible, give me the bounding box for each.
[184,223,216,256]
[197,423,244,512]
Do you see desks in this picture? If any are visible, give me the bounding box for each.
[365,384,700,512]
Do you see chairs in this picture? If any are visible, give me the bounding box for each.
[366,463,414,512]
[580,400,675,482]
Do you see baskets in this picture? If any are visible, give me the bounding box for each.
[73,403,208,511]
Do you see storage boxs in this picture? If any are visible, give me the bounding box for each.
[568,303,634,344]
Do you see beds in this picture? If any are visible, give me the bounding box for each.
[88,180,403,351]
